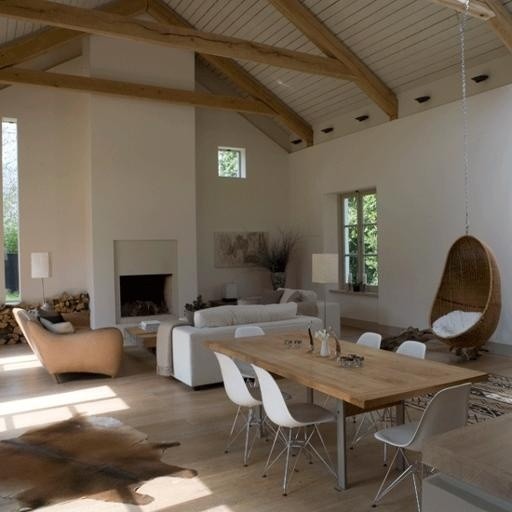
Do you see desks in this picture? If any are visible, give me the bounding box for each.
[209,330,491,493]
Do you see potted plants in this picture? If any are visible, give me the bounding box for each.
[261,226,304,299]
[184,295,207,322]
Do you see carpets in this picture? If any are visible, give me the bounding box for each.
[400,369,511,424]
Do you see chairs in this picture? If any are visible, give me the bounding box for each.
[249,362,337,498]
[429,235,502,352]
[395,339,427,360]
[210,350,292,456]
[356,332,382,350]
[372,381,475,507]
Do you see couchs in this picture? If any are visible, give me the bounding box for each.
[235,287,341,339]
[156,299,324,389]
[13,308,124,384]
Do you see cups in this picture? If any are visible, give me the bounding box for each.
[293,340,303,351]
[347,354,356,358]
[360,356,364,366]
[344,358,354,369]
[283,339,293,351]
[353,357,360,367]
[336,355,346,365]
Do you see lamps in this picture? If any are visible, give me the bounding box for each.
[311,252,340,303]
[29,251,50,279]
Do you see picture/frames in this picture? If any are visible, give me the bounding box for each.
[213,232,269,269]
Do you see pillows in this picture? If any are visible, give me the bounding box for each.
[39,316,75,335]
[255,289,282,303]
[286,291,302,302]
[36,308,65,323]
